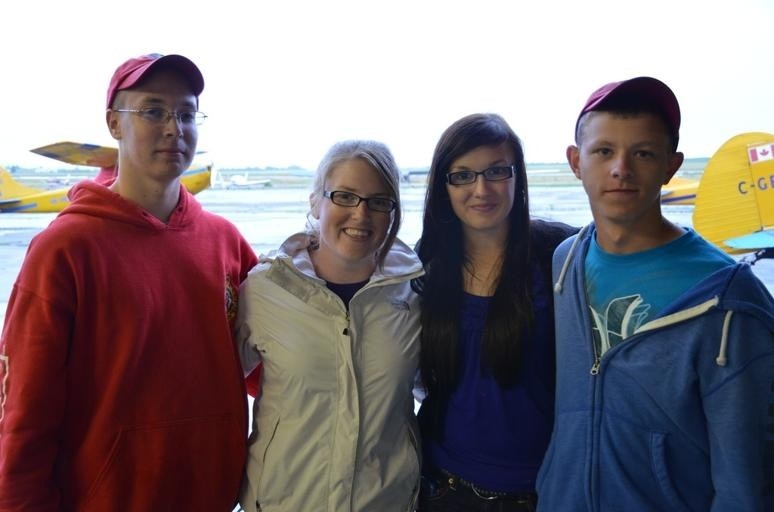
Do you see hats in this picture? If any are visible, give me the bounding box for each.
[575,76,680,152]
[106,53,204,108]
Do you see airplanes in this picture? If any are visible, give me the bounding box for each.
[0,140,271,212]
[661,132,774,262]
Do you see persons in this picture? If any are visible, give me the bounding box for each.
[0,52,265,512]
[235,139,425,512]
[536,77,774,512]
[409,112,582,512]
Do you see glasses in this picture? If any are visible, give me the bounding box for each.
[445,164,516,185]
[323,190,397,213]
[113,108,207,125]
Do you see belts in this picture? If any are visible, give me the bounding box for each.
[424,465,533,512]
